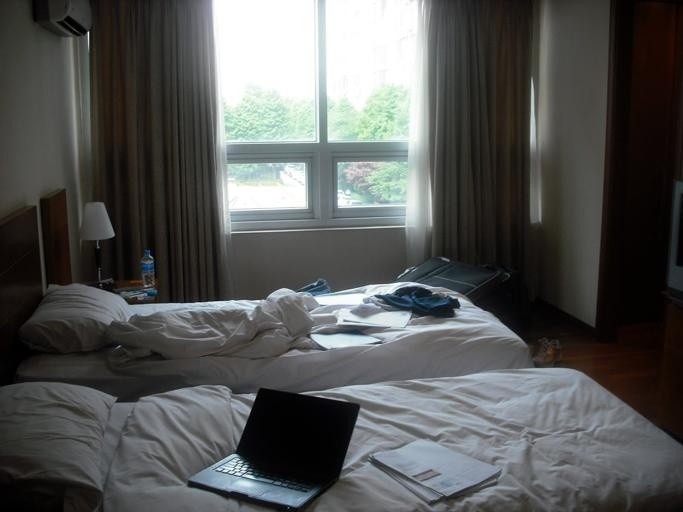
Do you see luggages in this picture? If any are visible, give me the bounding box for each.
[393,256,505,306]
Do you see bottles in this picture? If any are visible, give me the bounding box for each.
[139,249,155,288]
[553,339,561,361]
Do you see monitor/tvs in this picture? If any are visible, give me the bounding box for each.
[666,179,683,300]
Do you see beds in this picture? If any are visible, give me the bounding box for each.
[20,282,533,400]
[62,367,681,512]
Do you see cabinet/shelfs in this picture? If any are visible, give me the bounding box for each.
[662,296,683,349]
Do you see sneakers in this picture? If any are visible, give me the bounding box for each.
[532,338,561,367]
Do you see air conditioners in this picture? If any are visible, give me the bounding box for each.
[31,0,93,38]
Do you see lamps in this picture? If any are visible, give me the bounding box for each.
[82,201,116,292]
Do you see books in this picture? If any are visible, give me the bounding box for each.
[368,436,503,505]
[307,330,383,352]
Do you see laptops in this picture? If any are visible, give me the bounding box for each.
[187,388,360,510]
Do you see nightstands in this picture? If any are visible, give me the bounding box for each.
[90,279,160,304]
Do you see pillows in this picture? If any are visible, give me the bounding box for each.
[22,284,127,353]
[0,382,118,498]
